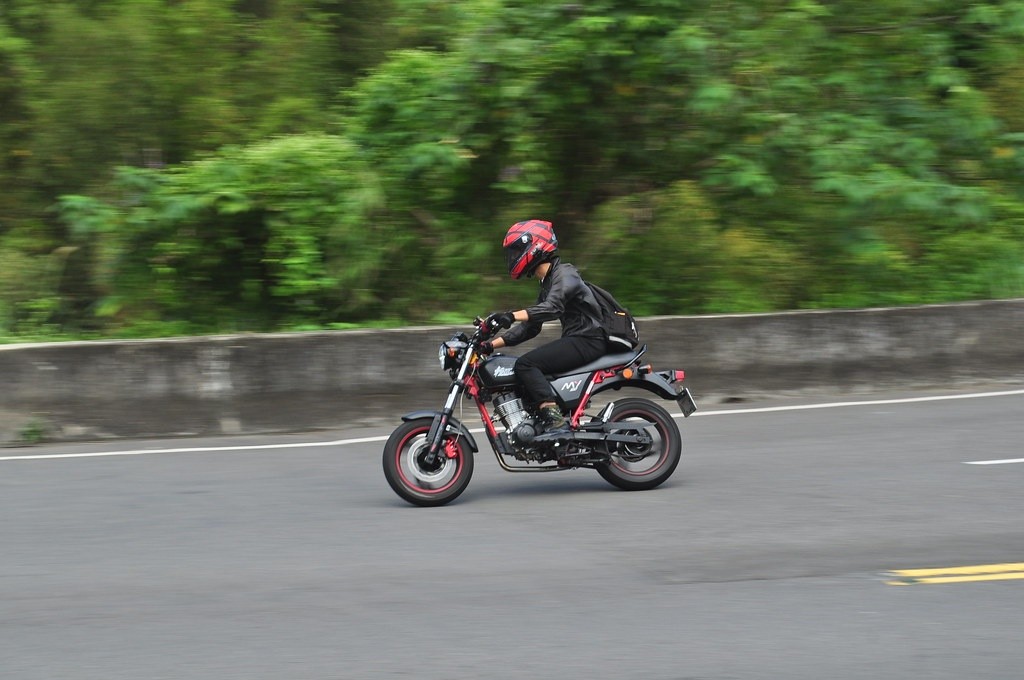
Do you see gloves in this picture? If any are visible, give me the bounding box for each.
[480,341,494,355]
[486,310,516,330]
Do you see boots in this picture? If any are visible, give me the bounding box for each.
[532,405,575,443]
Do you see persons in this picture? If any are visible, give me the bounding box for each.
[478,219,609,444]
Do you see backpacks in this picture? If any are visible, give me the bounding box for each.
[551,262,640,351]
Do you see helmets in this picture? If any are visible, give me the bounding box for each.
[502,219,559,281]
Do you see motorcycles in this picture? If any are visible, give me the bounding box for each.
[382,311,698,507]
